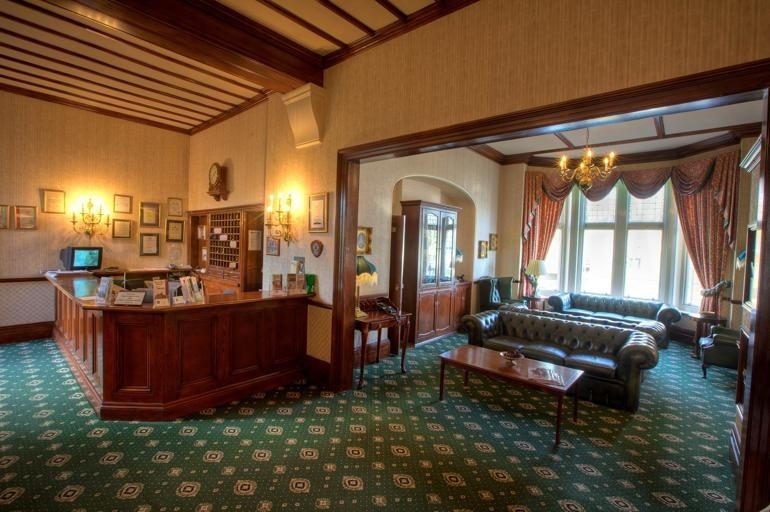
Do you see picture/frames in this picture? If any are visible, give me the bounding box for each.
[42,187,67,216]
[0,203,10,230]
[306,190,329,234]
[112,192,187,258]
[356,226,373,256]
[13,204,38,231]
[479,240,488,260]
[489,233,499,251]
[265,236,281,257]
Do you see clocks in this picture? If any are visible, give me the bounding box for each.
[208,161,221,188]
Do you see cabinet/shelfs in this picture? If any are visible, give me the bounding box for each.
[727,130,765,488]
[456,280,472,331]
[399,198,463,348]
[198,273,260,297]
[203,211,263,287]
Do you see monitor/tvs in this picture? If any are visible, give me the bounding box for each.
[61,247,103,271]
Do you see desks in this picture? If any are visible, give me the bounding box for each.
[355,305,413,392]
[690,315,728,360]
[437,344,588,446]
[523,295,552,310]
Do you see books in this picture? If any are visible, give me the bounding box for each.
[528,367,565,387]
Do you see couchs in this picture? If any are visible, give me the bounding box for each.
[499,301,667,346]
[457,307,659,415]
[545,290,683,348]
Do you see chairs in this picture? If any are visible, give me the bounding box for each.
[474,275,530,311]
[697,324,743,380]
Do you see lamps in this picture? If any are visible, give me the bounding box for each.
[263,190,297,248]
[355,253,379,318]
[555,127,619,195]
[526,259,549,297]
[69,193,113,242]
